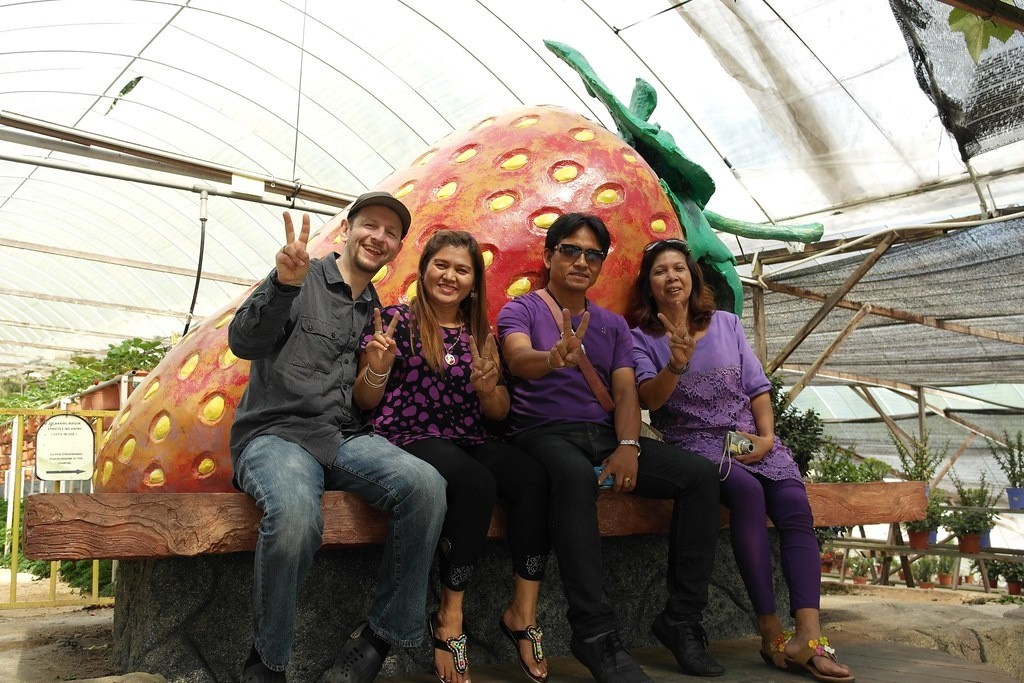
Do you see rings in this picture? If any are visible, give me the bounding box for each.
[472,354,480,358]
[283,245,286,253]
[624,478,631,484]
[481,354,491,358]
[575,333,584,339]
[677,320,686,326]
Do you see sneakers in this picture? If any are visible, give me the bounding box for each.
[651,612,724,676]
[569,621,653,683]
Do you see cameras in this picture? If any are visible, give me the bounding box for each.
[723,431,754,456]
[594,466,615,489]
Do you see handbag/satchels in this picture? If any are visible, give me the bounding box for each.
[640,421,666,442]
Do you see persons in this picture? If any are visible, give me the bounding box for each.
[229,193,447,683]
[497,213,726,683]
[631,238,856,683]
[353,229,551,683]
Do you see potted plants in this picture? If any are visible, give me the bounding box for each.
[1,339,169,484]
[806,428,1024,596]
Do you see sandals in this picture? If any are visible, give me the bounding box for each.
[242,643,287,683]
[322,619,383,683]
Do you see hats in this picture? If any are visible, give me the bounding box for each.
[348,191,411,240]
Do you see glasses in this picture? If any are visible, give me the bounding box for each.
[644,238,686,252]
[554,243,605,262]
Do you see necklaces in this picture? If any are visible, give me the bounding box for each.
[440,313,463,366]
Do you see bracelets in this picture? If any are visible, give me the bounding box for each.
[363,363,391,388]
[544,350,568,369]
[667,358,690,374]
[618,439,641,457]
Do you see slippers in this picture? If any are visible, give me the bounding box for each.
[786,637,855,683]
[498,615,550,683]
[759,631,795,672]
[429,611,471,683]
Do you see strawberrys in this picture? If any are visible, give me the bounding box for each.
[2,380,99,447]
[94,39,823,492]
